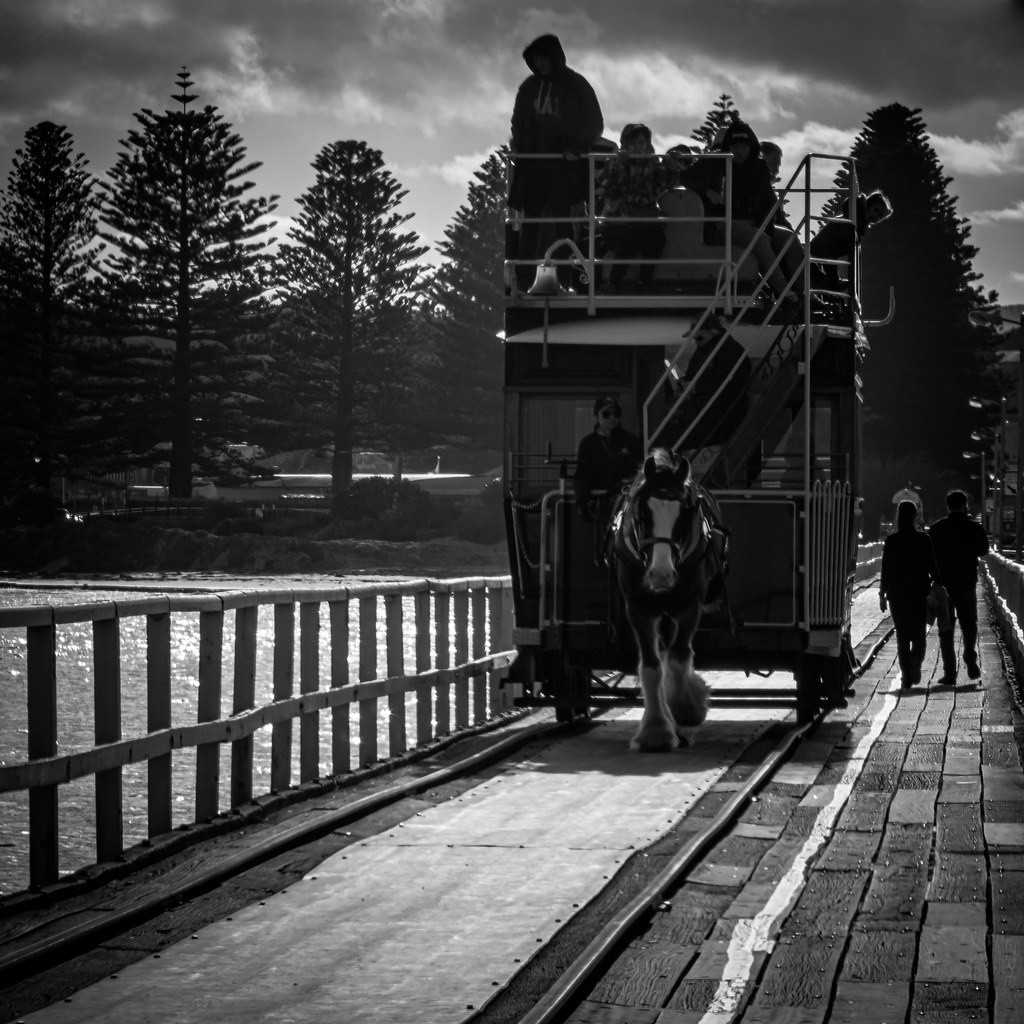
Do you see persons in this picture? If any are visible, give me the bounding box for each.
[880,502,943,695]
[668,312,752,482]
[602,122,892,324]
[506,34,603,295]
[930,490,989,685]
[573,395,642,522]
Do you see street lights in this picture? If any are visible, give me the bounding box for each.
[970,305,1024,564]
[969,395,1007,557]
[963,450,988,533]
[971,430,1001,552]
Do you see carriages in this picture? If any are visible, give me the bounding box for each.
[494,142,896,754]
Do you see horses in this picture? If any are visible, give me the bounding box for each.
[613,446,729,751]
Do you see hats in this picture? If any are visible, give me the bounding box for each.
[594,395,625,413]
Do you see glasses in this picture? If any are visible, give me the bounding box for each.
[600,409,620,420]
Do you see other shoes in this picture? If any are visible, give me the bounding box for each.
[901,671,914,690]
[936,674,959,688]
[963,650,980,679]
[910,655,926,683]
